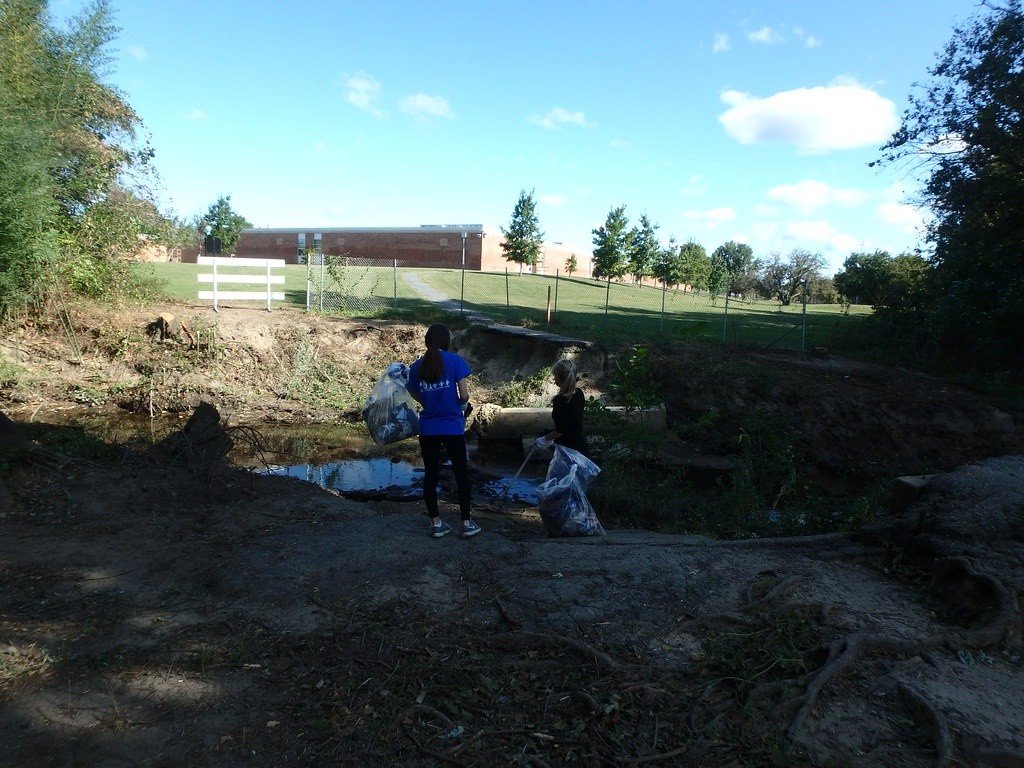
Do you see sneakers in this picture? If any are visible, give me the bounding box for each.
[434,519,452,536]
[462,519,481,536]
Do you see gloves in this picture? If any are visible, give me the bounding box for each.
[398,368,409,386]
[459,399,468,411]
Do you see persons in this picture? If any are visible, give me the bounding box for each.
[545,358,586,453]
[405,323,481,537]
